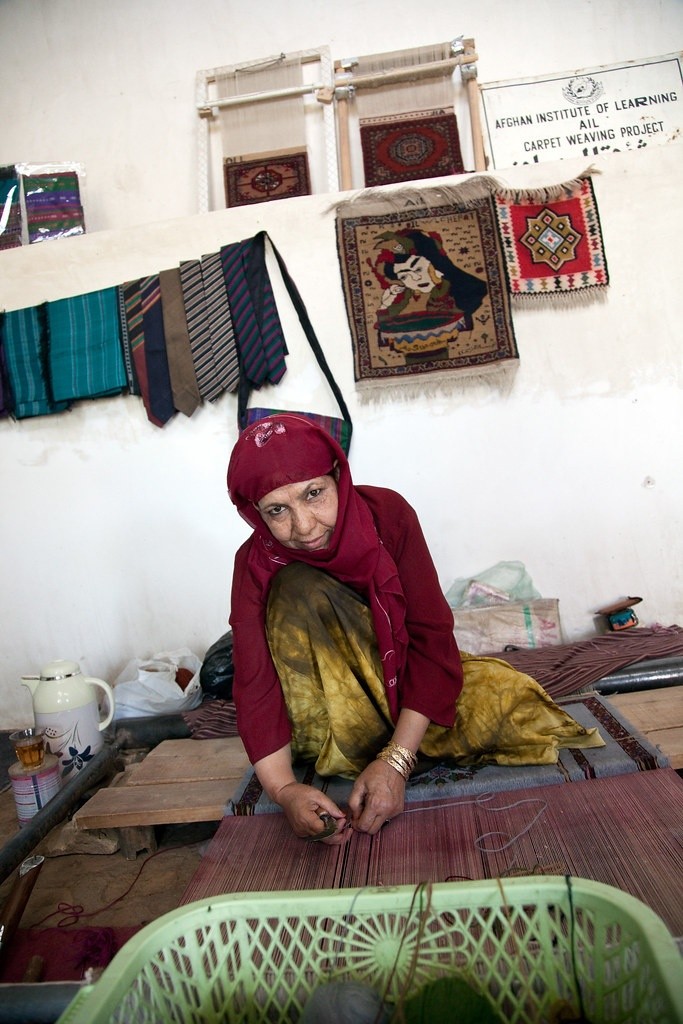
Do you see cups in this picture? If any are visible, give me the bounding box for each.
[9,726,50,772]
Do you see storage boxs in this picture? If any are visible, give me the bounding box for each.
[58,876,683,1024]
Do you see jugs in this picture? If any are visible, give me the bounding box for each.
[20,660,115,787]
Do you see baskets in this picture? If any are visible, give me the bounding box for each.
[54,875,683,1024]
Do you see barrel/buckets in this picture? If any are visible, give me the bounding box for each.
[8,754,64,828]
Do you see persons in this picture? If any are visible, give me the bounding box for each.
[227,414,605,848]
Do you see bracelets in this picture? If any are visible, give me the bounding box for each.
[377,741,418,780]
